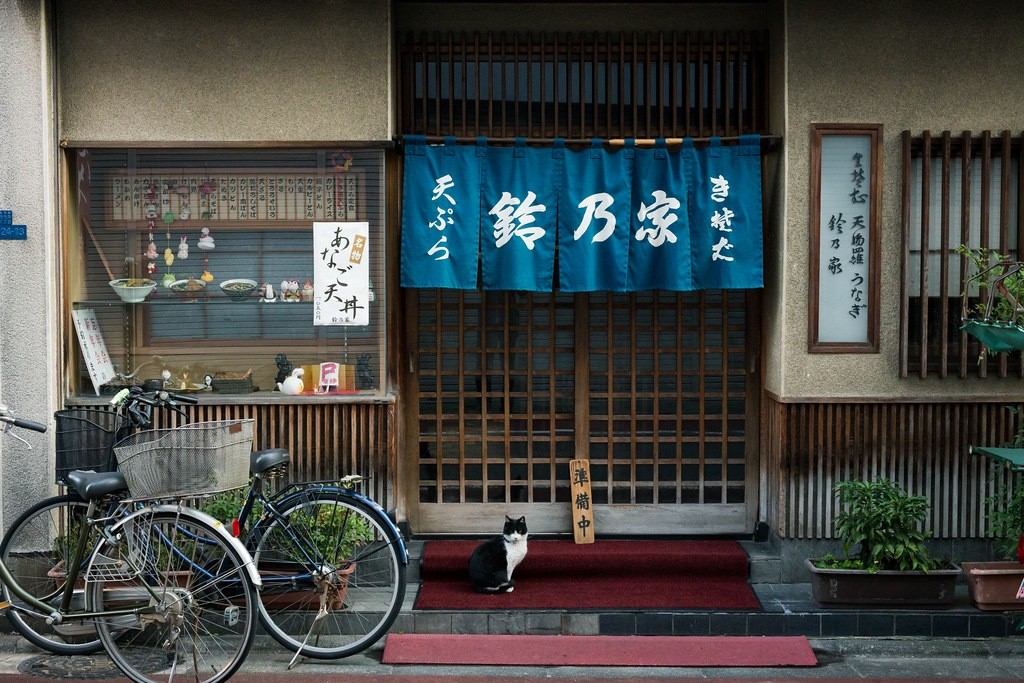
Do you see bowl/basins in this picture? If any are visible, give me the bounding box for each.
[109,279,157,302]
[145,379,163,391]
[169,279,206,302]
[219,279,257,300]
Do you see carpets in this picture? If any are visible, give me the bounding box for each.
[382,634,820,667]
[411,535,763,611]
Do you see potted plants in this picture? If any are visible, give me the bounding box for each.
[955,244,1024,365]
[203,476,374,610]
[46,505,199,609]
[961,405,1024,611]
[803,475,964,607]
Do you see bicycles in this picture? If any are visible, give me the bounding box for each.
[0,385,411,660]
[0,404,263,683]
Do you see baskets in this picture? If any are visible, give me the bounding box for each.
[54,409,128,488]
[113,418,254,500]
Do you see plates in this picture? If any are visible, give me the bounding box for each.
[163,382,207,391]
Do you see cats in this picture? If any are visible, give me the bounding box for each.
[466,514,529,594]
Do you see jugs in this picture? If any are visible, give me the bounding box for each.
[277,376,304,394]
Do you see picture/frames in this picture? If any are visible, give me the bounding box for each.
[809,122,885,355]
